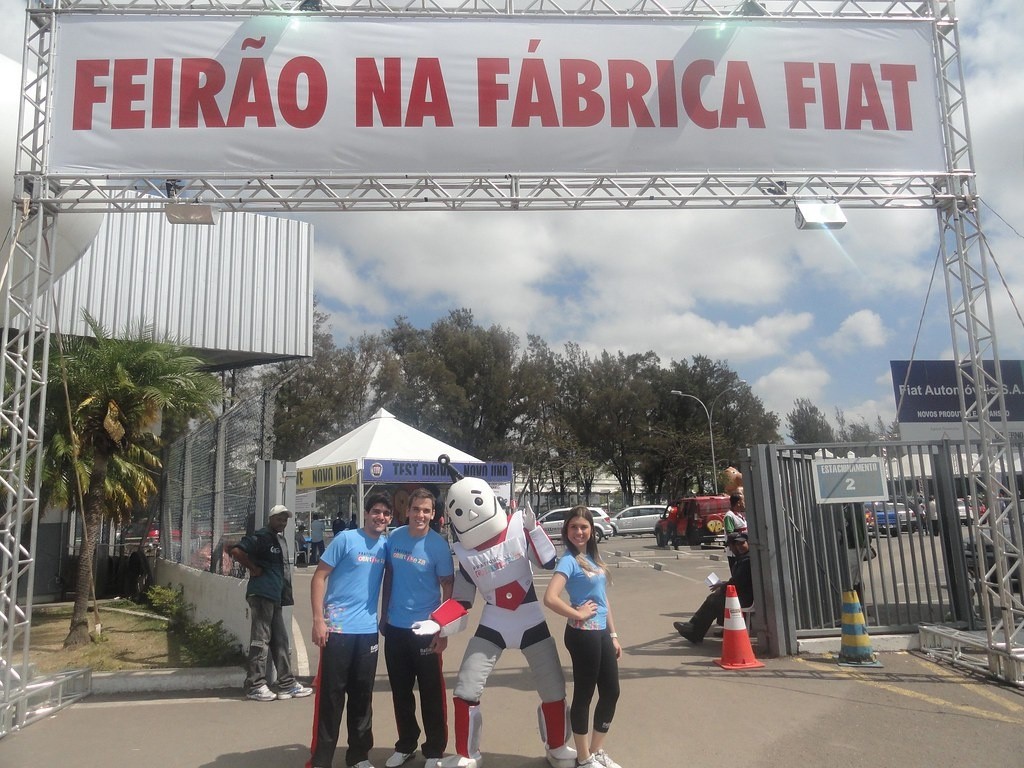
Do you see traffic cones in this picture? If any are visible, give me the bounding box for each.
[833,589,884,669]
[713,585,765,671]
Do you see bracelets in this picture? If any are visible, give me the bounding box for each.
[610,633,617,638]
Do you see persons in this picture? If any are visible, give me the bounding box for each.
[295,525,309,562]
[333,511,357,536]
[311,514,325,563]
[723,491,747,569]
[232,505,313,702]
[306,491,393,768]
[411,454,577,768]
[673,532,754,645]
[543,506,622,768]
[918,495,939,536]
[379,488,454,768]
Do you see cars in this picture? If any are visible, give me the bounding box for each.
[610,504,673,538]
[862,501,917,537]
[956,496,987,526]
[963,498,1024,593]
[535,506,613,543]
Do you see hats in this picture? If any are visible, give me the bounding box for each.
[734,531,749,541]
[268,503,293,518]
[722,532,738,546]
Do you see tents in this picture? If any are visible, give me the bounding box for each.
[294,407,482,529]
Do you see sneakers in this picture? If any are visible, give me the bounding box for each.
[245,683,278,702]
[424,757,443,768]
[276,681,314,700]
[575,753,607,768]
[712,628,723,637]
[384,748,417,768]
[351,760,375,768]
[595,749,622,768]
[673,621,703,645]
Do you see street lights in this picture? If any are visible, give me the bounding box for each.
[671,379,749,496]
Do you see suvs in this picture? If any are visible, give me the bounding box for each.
[654,492,732,548]
